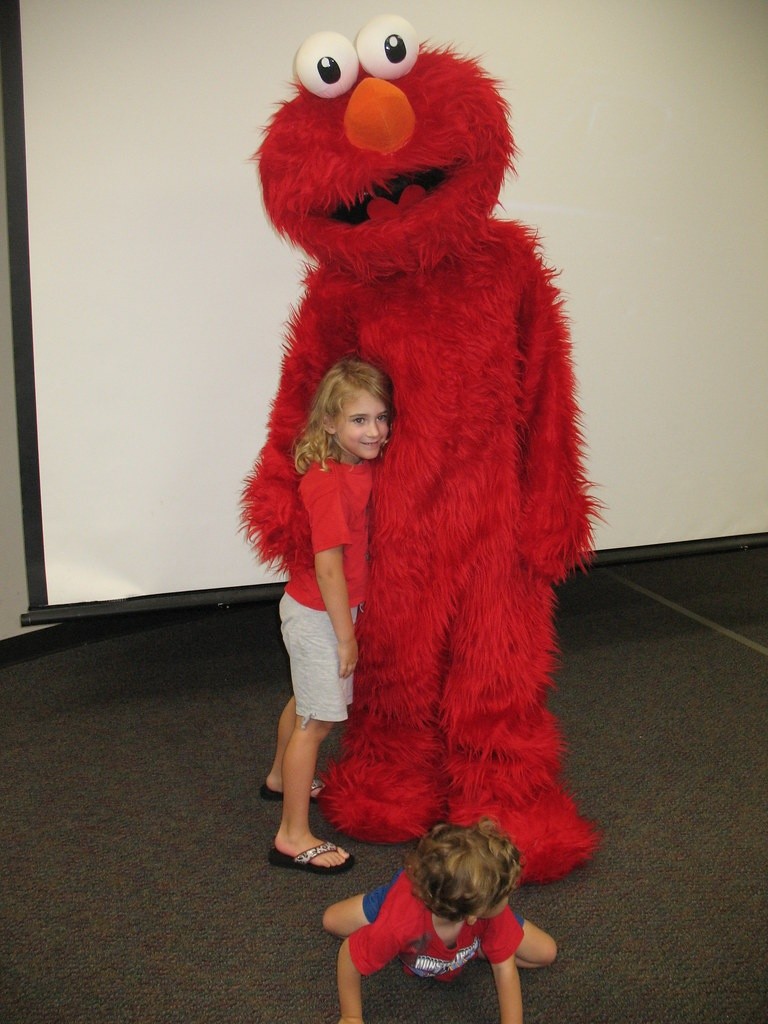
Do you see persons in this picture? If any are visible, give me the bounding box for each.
[323,816,559,1024]
[259,355,396,877]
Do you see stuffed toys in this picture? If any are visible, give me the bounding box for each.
[243,15,607,887]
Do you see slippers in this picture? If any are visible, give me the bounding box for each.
[270,841,354,874]
[260,778,326,804]
